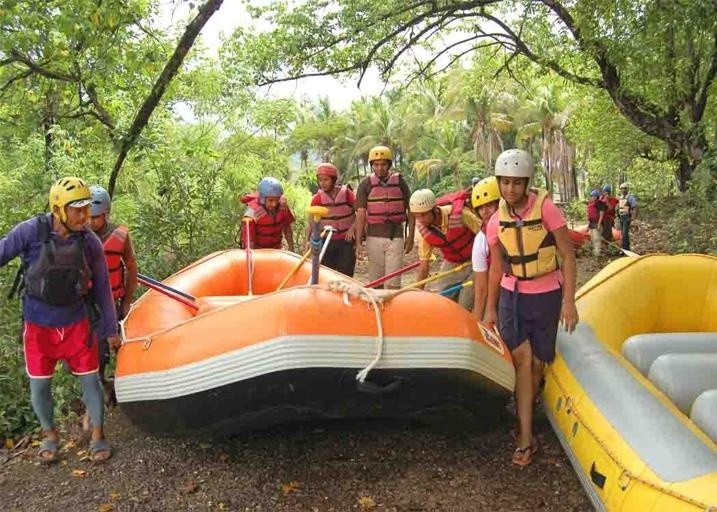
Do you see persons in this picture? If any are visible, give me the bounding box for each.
[482,148,580,466]
[306,161,359,279]
[353,146,417,290]
[470,175,503,320]
[0,175,123,464]
[239,176,295,255]
[466,175,480,191]
[408,185,482,311]
[585,181,638,260]
[85,185,137,409]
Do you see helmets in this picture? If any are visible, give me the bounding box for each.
[591,183,629,196]
[369,145,392,162]
[89,185,111,220]
[471,176,501,209]
[316,162,338,177]
[258,177,283,205]
[409,189,437,213]
[494,148,535,196]
[48,176,91,223]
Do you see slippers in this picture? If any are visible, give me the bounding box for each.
[88,442,113,463]
[512,446,534,466]
[37,442,61,462]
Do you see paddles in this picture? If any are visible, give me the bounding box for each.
[600,235,638,257]
[405,262,472,287]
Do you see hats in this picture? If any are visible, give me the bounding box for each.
[67,198,94,208]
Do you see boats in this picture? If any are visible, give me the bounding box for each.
[108,247,518,439]
[567,223,622,244]
[539,249,717,512]
